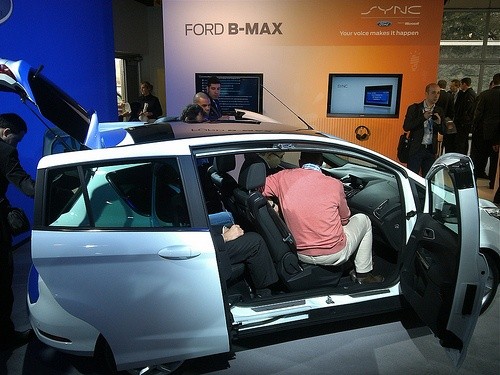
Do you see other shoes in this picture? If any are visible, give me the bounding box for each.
[358,272,386,283]
[259,284,287,298]
[489,183,494,189]
[477,172,488,179]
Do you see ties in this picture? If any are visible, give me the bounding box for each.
[452,93,455,104]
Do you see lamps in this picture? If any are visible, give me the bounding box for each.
[440,40,500,46]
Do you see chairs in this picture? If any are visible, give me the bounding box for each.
[207,154,240,210]
[231,153,344,291]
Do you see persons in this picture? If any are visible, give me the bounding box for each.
[239,151,286,206]
[437,73,500,206]
[176,77,223,123]
[125,168,285,298]
[0,112,64,352]
[249,150,384,284]
[117,80,163,122]
[403,83,447,182]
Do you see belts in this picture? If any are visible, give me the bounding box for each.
[423,144,433,149]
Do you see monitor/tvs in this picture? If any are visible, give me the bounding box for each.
[326,74,403,118]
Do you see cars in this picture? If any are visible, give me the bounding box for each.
[0,57,500,375]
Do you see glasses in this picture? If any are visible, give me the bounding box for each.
[273,153,284,159]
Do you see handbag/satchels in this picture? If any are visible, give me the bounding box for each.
[397,134,411,163]
[8,207,30,237]
